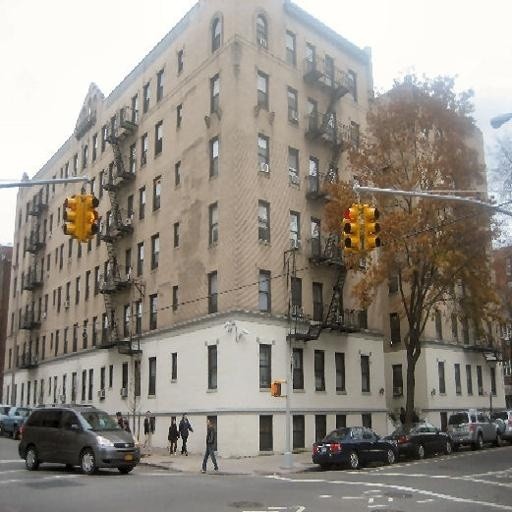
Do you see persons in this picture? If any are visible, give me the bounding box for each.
[116,411,132,433]
[168,415,181,456]
[143,411,156,451]
[179,412,194,457]
[200,418,220,474]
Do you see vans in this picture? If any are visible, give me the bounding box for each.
[18,403,141,478]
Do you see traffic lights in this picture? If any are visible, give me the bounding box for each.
[270,381,282,398]
[62,193,83,242]
[339,219,352,256]
[359,202,381,251]
[78,192,99,242]
[342,203,361,253]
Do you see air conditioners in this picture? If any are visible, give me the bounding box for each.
[259,162,269,173]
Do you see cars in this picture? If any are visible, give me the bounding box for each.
[308,407,512,472]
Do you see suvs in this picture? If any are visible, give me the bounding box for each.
[0,404,34,441]
